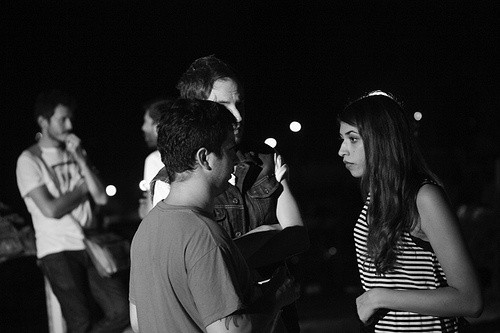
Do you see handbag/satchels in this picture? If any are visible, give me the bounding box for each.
[82,232,131,278]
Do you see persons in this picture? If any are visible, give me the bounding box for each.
[16,102,130,333]
[150,54,309,333]
[130,99,301,333]
[339,91,483,333]
[139,102,164,218]
